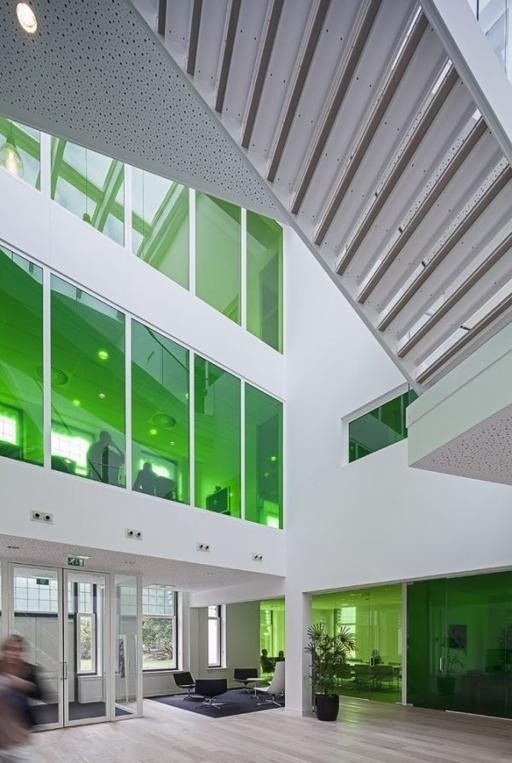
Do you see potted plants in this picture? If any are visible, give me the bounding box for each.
[303,621,355,722]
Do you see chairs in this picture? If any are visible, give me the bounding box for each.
[0,439,178,502]
[335,658,402,689]
[173,661,285,710]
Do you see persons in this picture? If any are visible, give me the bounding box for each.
[134,463,157,495]
[277,651,285,661]
[86,430,124,486]
[261,649,274,673]
[0,635,43,763]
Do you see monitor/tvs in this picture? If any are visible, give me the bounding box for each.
[205,487,230,514]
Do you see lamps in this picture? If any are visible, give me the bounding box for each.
[0,121,25,179]
